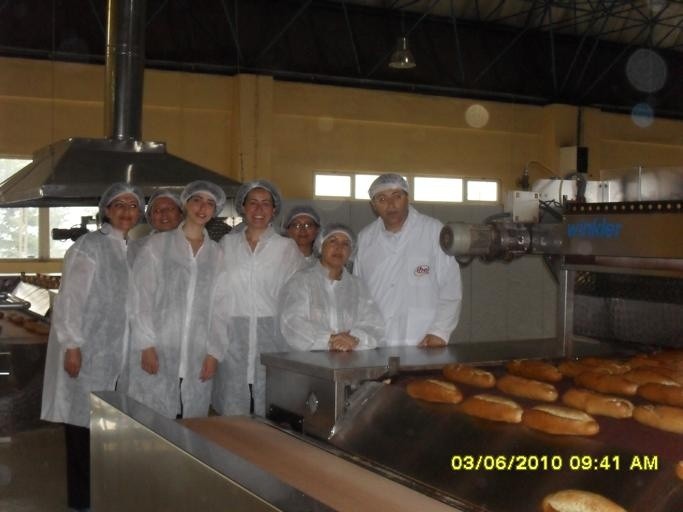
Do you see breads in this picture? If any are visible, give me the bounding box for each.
[558,351,683,395]
[442,363,497,388]
[462,392,524,423]
[496,374,559,402]
[562,388,634,419]
[521,404,600,435]
[405,377,463,404]
[503,359,562,382]
[632,404,683,435]
[636,382,683,409]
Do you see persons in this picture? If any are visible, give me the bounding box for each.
[123,179,236,419]
[277,223,387,351]
[39,182,145,511]
[125,188,187,270]
[279,205,327,270]
[351,173,462,348]
[209,179,313,418]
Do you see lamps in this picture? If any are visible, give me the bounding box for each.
[388,1,417,67]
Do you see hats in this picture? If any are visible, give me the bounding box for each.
[99,181,147,225]
[234,180,283,224]
[281,205,322,233]
[146,188,186,229]
[180,179,228,219]
[313,223,359,259]
[368,173,410,199]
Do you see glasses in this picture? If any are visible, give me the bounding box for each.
[326,238,352,248]
[291,221,315,230]
[112,198,139,210]
[153,206,177,213]
[375,194,402,202]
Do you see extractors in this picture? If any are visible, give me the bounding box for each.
[1,0,242,208]
